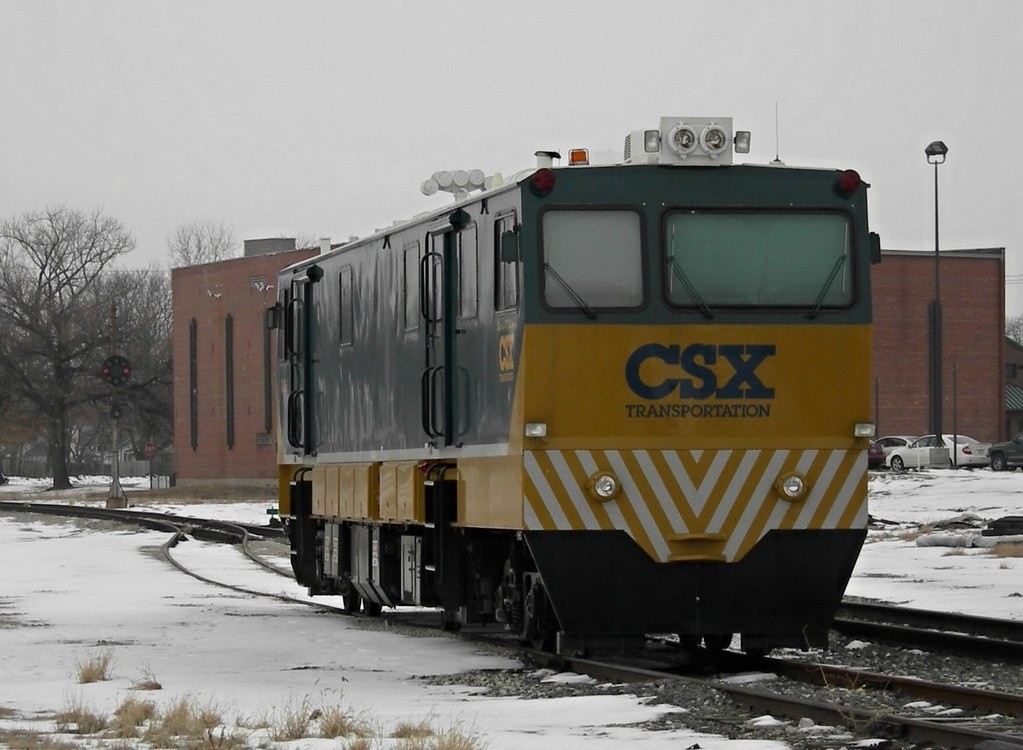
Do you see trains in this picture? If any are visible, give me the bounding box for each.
[264,115,884,673]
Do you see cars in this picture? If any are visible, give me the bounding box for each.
[874,435,919,470]
[869,439,886,470]
[886,434,991,472]
[987,430,1023,472]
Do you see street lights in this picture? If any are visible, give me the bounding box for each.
[923,140,949,444]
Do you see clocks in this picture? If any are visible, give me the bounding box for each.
[102,356,131,386]
